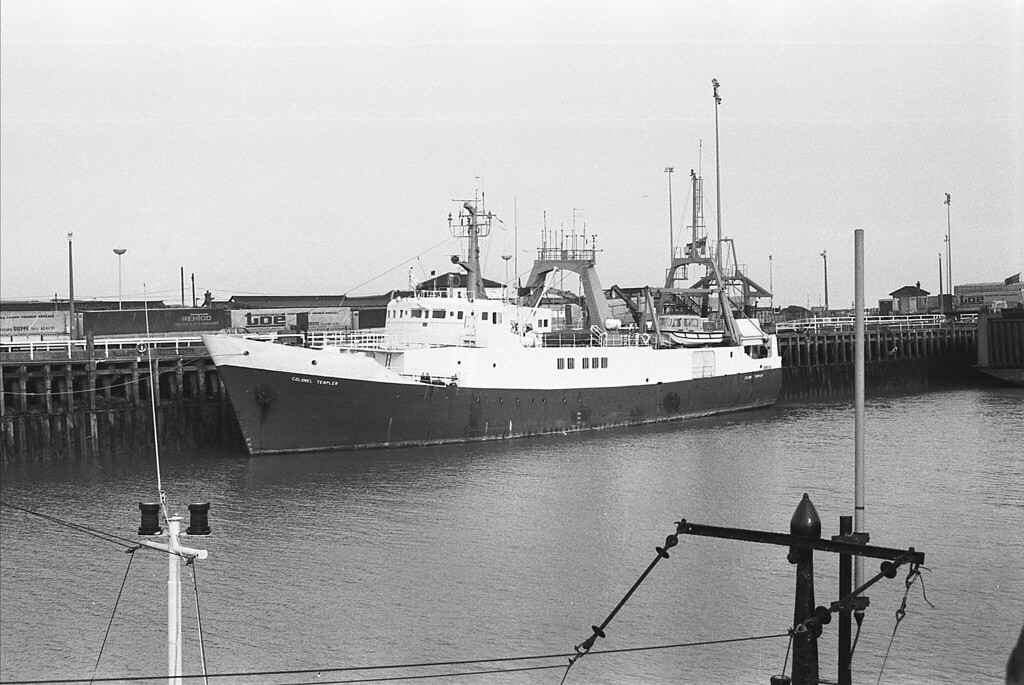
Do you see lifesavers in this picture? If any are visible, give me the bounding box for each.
[135,341,148,353]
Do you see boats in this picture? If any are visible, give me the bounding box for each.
[199,79,782,455]
